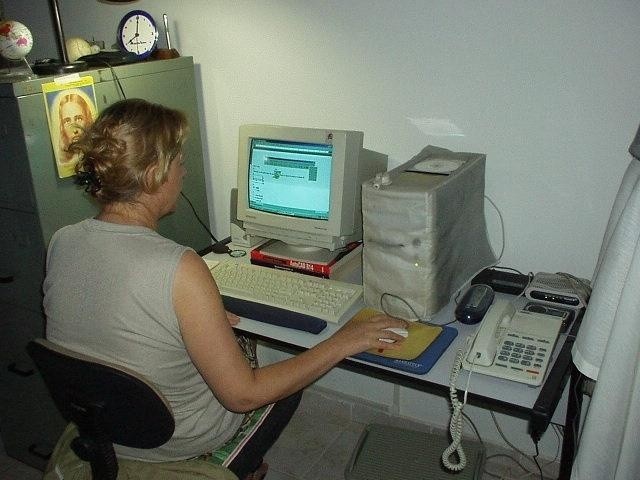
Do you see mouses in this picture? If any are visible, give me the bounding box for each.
[378,327,409,343]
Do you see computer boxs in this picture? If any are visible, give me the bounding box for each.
[362,144,487,317]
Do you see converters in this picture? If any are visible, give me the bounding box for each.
[470,268,534,295]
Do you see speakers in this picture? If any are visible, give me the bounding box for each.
[230,188,266,248]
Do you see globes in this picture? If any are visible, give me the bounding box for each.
[1,21,34,75]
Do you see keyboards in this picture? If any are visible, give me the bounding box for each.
[209,258,363,326]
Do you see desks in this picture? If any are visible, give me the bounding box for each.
[196,236,593,479]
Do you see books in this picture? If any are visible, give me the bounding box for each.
[250,238,363,280]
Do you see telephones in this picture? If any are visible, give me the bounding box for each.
[462,301,570,386]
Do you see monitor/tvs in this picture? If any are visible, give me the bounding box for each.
[236,124,388,266]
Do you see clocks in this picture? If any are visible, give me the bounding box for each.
[117,9,159,61]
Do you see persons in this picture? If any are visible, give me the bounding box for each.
[43,99,410,480]
[50,90,95,165]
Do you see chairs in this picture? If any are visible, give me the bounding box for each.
[27,335,304,479]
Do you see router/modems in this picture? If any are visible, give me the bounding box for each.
[525,271,591,309]
